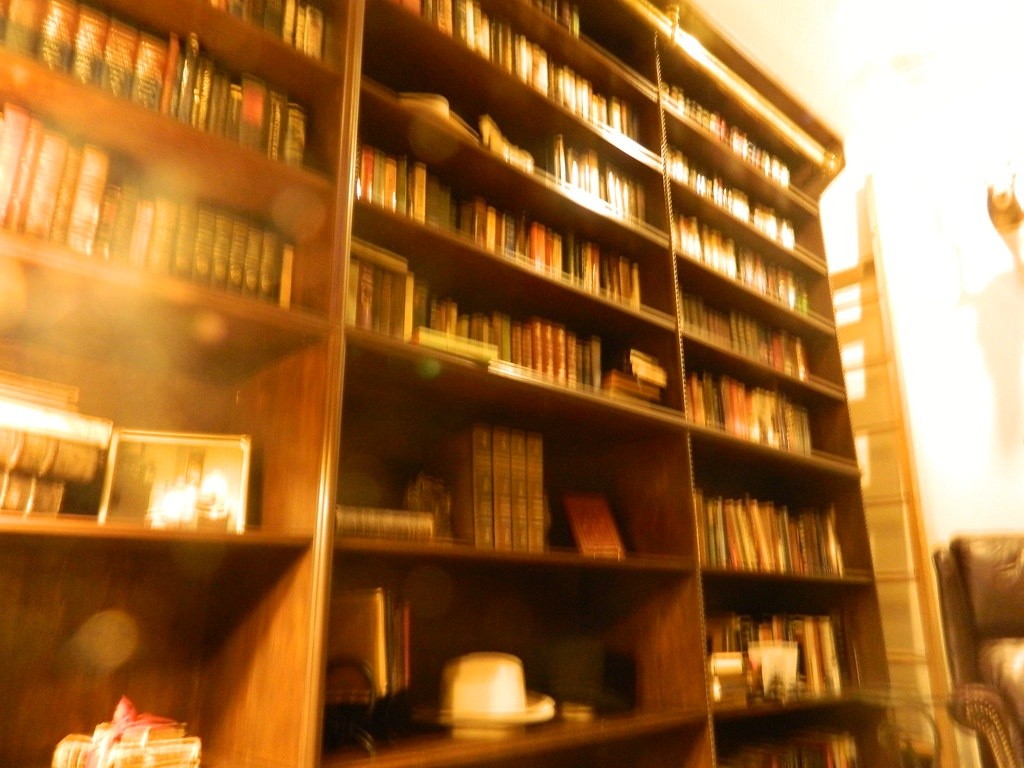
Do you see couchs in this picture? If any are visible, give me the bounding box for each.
[932,533,1024,767]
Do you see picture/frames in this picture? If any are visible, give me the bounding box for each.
[97,427,251,534]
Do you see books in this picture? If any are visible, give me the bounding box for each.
[0,100,295,308]
[325,586,410,703]
[0,369,115,514]
[663,83,789,189]
[346,233,668,401]
[707,606,863,702]
[689,369,812,456]
[399,90,647,220]
[672,205,808,316]
[354,135,641,310]
[531,0,581,35]
[391,0,520,78]
[520,35,641,145]
[696,485,844,578]
[682,294,806,381]
[0,0,305,168]
[333,419,625,557]
[210,0,332,64]
[717,727,857,768]
[667,143,796,251]
[123,722,187,739]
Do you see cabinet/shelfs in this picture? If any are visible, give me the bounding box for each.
[1,0,906,768]
